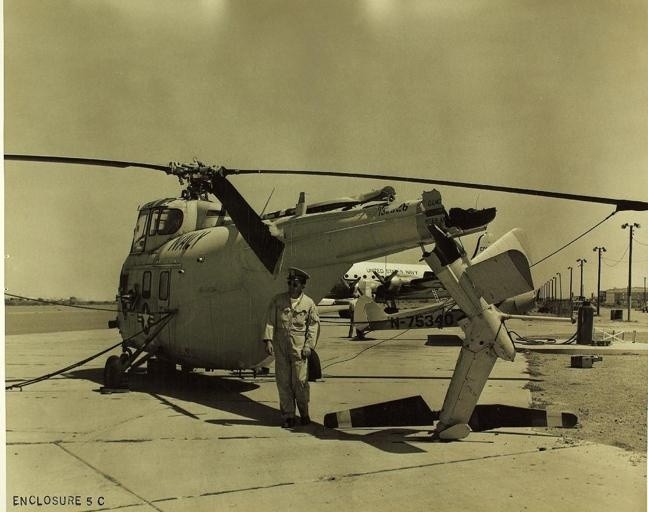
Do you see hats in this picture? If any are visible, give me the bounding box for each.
[286,266,309,280]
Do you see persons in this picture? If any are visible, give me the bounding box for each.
[262,266,334,428]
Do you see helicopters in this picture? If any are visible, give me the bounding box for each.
[3,153,648,440]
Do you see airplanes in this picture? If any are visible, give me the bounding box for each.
[318,257,435,300]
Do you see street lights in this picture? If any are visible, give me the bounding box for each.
[535,265,572,306]
[592,246,607,314]
[576,258,587,297]
[622,222,641,322]
